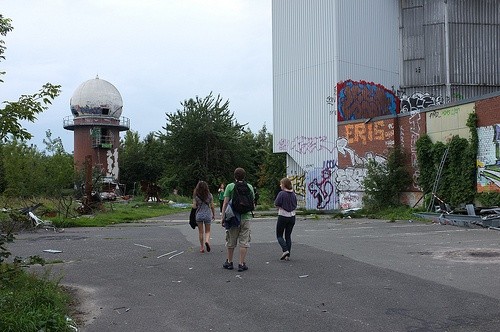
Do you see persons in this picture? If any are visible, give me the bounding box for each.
[218,183,226,215]
[221,168,255,271]
[192,181,215,252]
[250,187,259,218]
[274,178,297,261]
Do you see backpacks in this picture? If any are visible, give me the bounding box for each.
[231,179,254,215]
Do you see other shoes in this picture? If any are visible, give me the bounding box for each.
[205,242,210,252]
[280,250,290,260]
[238,262,249,271]
[222,259,233,269]
[199,249,204,253]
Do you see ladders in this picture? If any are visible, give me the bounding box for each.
[427,149,449,212]
